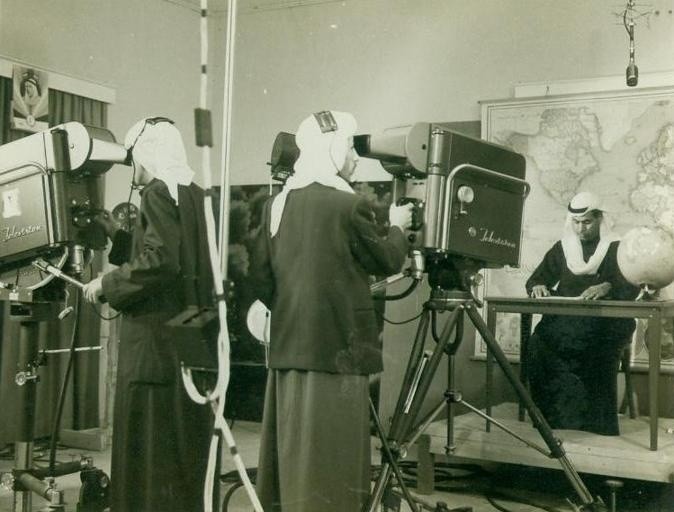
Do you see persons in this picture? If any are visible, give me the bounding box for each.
[525,192,636,436]
[253,110,414,512]
[83,116,220,512]
[22,78,41,106]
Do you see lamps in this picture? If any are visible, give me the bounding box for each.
[622,0,638,87]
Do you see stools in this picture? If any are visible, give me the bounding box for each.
[617,347,637,419]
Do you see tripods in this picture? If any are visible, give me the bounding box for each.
[365,288,609,512]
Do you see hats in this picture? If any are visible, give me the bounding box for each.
[568,192,607,218]
[124,117,186,169]
[295,111,358,150]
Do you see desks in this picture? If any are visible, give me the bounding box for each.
[483,296,674,450]
